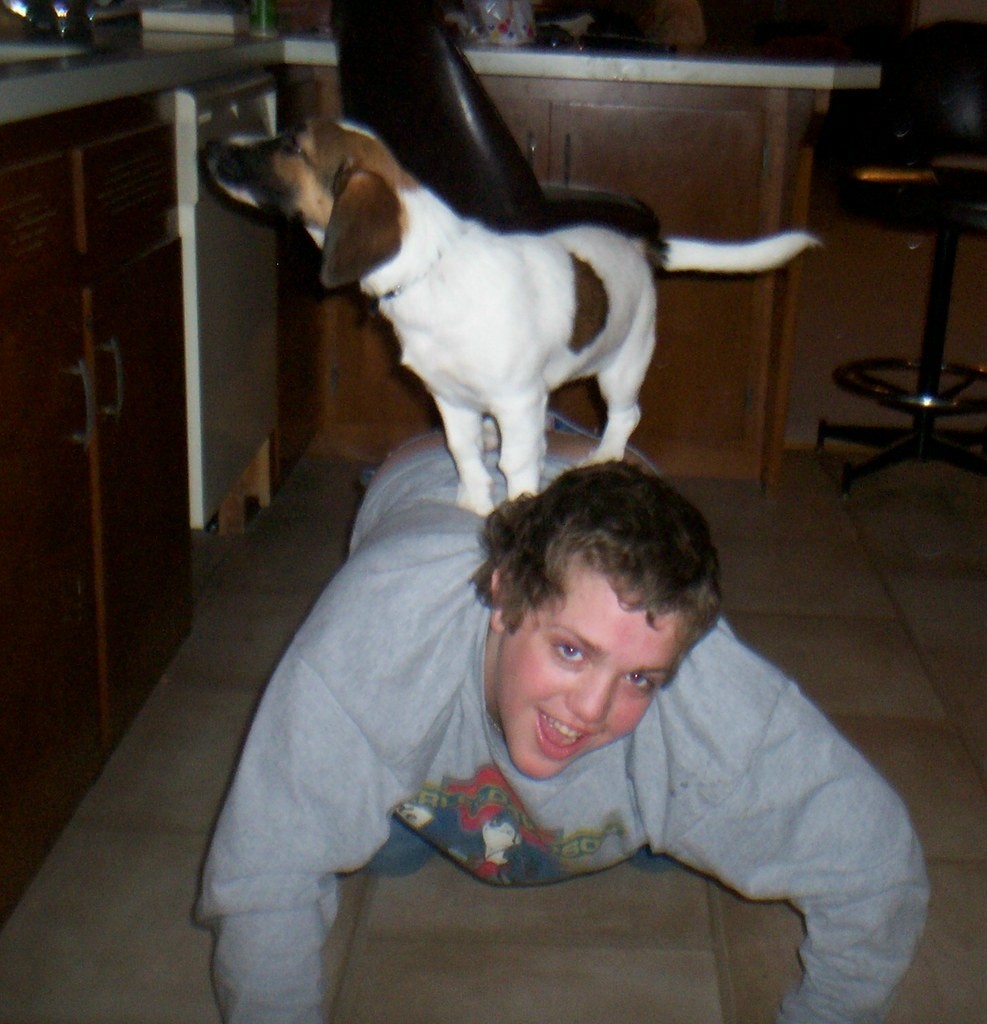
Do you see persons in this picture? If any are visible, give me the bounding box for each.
[196,415,930,1024]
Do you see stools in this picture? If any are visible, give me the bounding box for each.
[818,18,986,502]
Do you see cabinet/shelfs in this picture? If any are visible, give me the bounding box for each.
[278,66,831,496]
[0,87,194,929]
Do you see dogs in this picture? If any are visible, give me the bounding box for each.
[206,116,822,518]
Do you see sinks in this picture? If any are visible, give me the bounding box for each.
[0,42,91,80]
